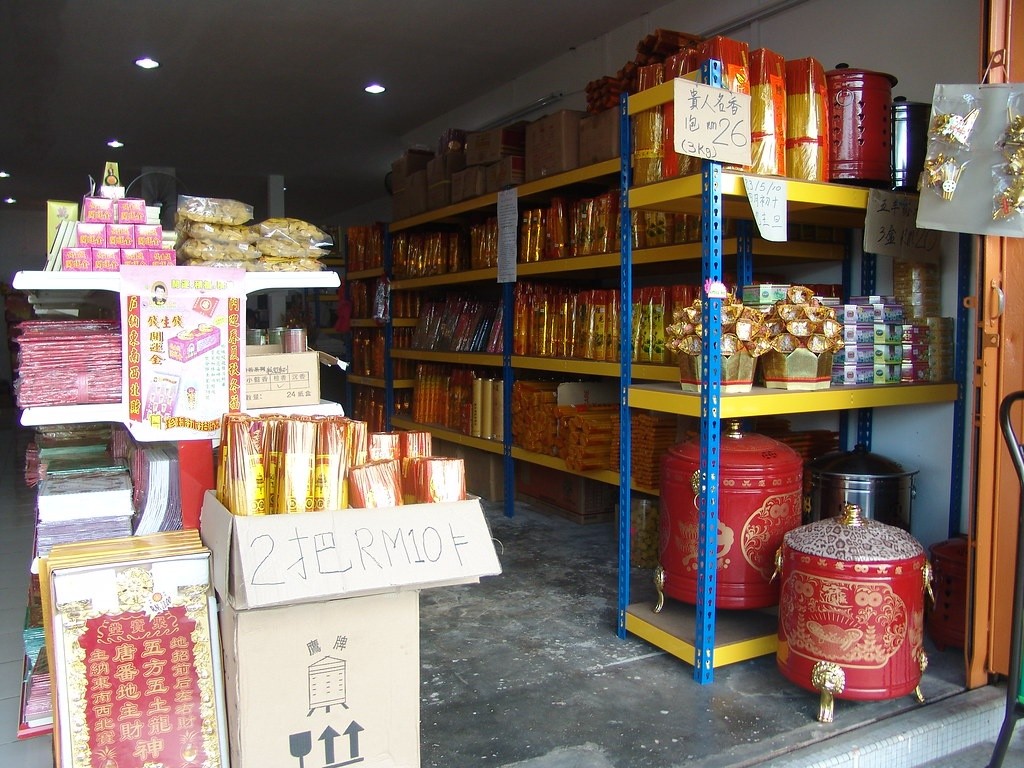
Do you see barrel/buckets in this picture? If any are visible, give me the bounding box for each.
[657,419,803,609]
[824,63,898,188]
[801,443,919,533]
[891,96,932,193]
[775,503,932,699]
[927,533,976,649]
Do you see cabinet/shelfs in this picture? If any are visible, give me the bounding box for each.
[344,221,417,420]
[383,156,852,519]
[303,224,345,335]
[11,270,341,768]
[616,61,973,685]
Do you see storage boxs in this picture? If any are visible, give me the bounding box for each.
[525,110,590,183]
[47,199,79,254]
[247,344,349,410]
[465,125,524,167]
[117,197,147,224]
[440,440,457,457]
[407,169,427,216]
[77,222,106,248]
[485,155,524,192]
[134,225,163,250]
[743,285,931,387]
[217,591,426,768]
[451,165,486,204]
[426,152,465,210]
[391,153,429,195]
[82,196,114,223]
[390,189,412,223]
[62,247,176,272]
[578,106,634,166]
[455,445,516,503]
[517,459,615,527]
[200,489,503,612]
[105,223,136,249]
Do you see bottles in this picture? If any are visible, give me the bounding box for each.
[285,316,307,353]
